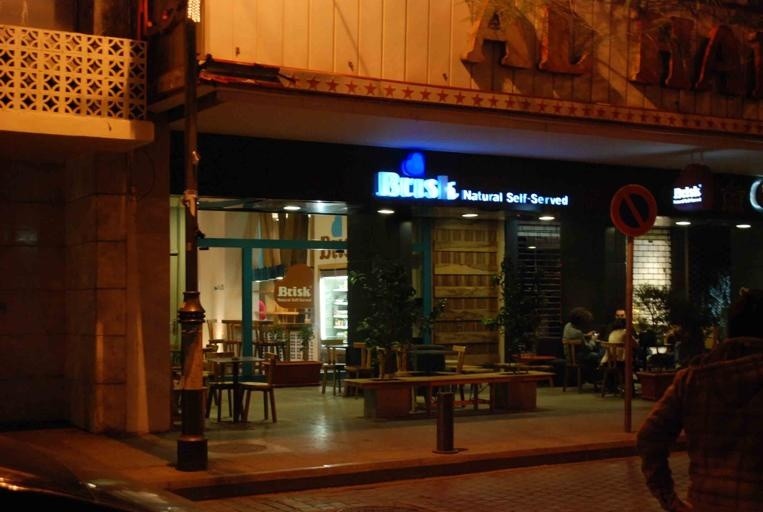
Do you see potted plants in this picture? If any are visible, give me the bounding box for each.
[483,258,558,368]
[636,287,679,401]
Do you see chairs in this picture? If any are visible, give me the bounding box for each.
[599,342,625,398]
[167,318,287,425]
[320,339,468,418]
[562,339,586,394]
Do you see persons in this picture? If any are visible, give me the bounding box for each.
[636,287,763,511]
[563,305,720,398]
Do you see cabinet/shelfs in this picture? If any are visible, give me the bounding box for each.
[331,287,348,351]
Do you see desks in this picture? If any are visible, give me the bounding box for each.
[342,370,557,421]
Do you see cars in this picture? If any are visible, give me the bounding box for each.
[0,428,217,512]
